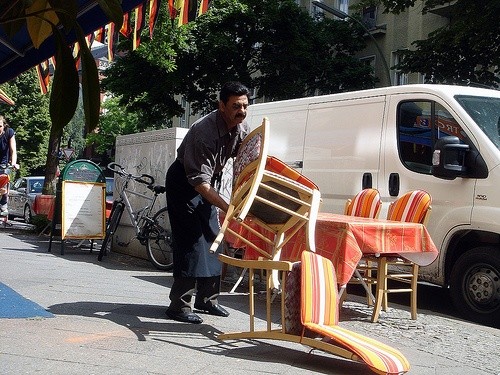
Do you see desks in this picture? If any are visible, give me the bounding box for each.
[31,195,113,248]
[217,211,440,323]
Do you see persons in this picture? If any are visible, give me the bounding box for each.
[165,80,250,325]
[0,115,20,176]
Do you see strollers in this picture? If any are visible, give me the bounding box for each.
[0,161,16,230]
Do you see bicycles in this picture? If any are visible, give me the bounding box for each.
[97,161,174,271]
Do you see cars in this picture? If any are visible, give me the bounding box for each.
[7,176,46,224]
[28,165,46,176]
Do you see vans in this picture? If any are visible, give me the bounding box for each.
[220,83,500,328]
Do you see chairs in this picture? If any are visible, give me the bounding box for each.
[213,250,412,375]
[347,189,433,324]
[208,117,321,293]
[344,188,389,312]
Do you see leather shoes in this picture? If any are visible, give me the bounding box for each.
[167,312,203,324]
[194,304,229,317]
